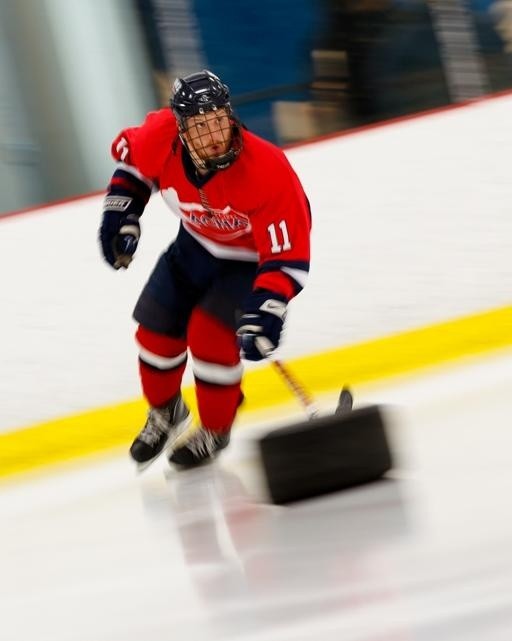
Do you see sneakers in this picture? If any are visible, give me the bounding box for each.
[129,392,190,462]
[168,427,230,468]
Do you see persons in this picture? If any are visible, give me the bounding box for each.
[98,70,313,468]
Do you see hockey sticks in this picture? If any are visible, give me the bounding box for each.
[255,335,353,423]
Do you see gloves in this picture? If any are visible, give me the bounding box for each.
[236,288,288,360]
[100,184,146,270]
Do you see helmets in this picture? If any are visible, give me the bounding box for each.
[168,68,243,172]
